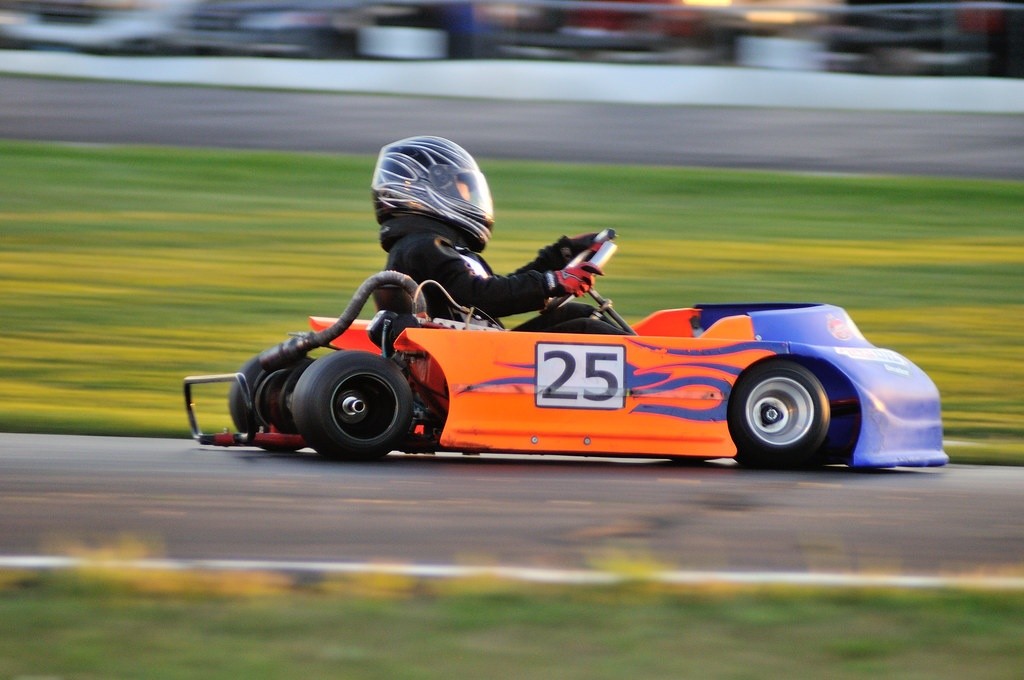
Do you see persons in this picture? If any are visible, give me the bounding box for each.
[373,134,627,334]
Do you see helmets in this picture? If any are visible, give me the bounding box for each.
[373,135,494,253]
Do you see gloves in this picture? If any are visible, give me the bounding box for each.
[543,261,604,296]
[556,233,598,267]
